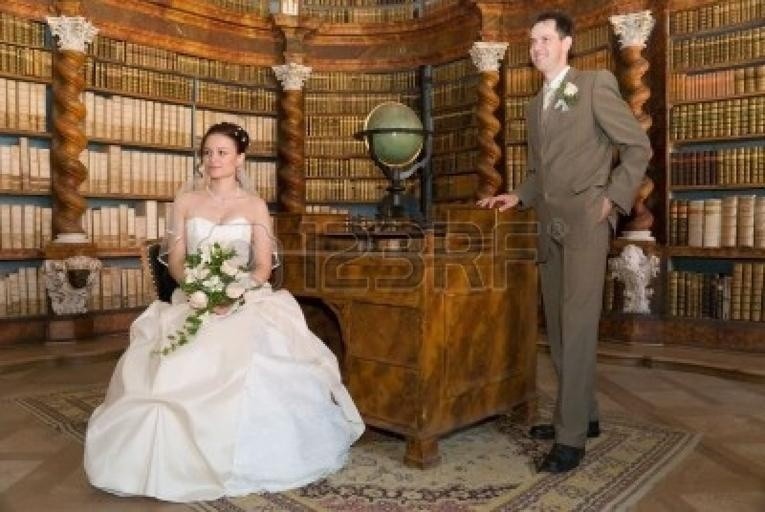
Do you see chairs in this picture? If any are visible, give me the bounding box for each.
[146,243,178,304]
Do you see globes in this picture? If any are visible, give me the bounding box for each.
[353,102,437,219]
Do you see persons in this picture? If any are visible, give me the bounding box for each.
[482,11,652,474]
[85,122,367,503]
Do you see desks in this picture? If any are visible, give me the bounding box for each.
[242,210,542,469]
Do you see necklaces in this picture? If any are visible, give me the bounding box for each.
[204,186,241,211]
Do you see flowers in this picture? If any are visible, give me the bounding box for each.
[551,83,579,115]
[155,242,249,356]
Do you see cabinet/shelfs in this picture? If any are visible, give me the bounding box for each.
[0,2,764,352]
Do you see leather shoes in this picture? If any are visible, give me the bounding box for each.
[538,444,585,472]
[530,420,599,439]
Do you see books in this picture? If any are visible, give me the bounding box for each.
[249,115,279,156]
[507,99,532,120]
[505,68,544,97]
[82,200,170,248]
[192,57,279,112]
[670,65,765,102]
[355,140,366,200]
[669,96,765,137]
[355,71,364,132]
[569,49,617,73]
[82,89,195,148]
[304,68,355,214]
[84,38,194,105]
[196,110,204,148]
[0,44,53,78]
[367,155,390,200]
[175,154,194,193]
[503,38,545,68]
[84,144,173,197]
[669,27,765,68]
[668,263,765,322]
[430,150,481,176]
[668,0,765,35]
[507,122,528,145]
[429,77,480,112]
[0,133,51,193]
[670,195,765,249]
[0,262,50,317]
[86,267,152,311]
[426,57,485,83]
[402,179,421,198]
[570,23,611,55]
[668,145,765,187]
[431,173,482,202]
[431,111,481,133]
[0,79,47,132]
[1,201,53,249]
[208,111,249,132]
[507,147,529,193]
[431,129,481,156]
[0,10,51,50]
[364,72,422,115]
[603,270,625,311]
[220,0,460,23]
[244,160,277,203]
